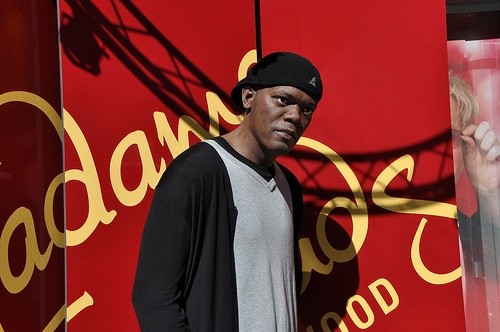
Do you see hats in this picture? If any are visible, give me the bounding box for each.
[231,51,322,116]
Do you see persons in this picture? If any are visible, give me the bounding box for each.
[450,74,500,331]
[128,52,323,331]
